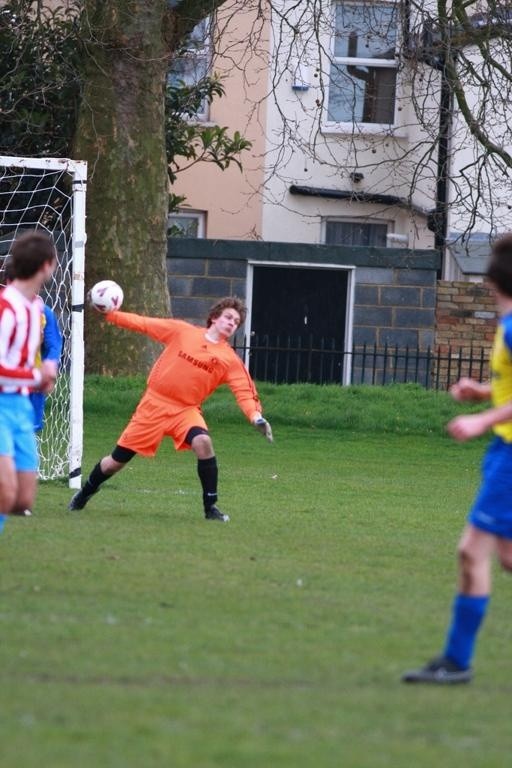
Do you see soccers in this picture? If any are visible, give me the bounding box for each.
[90,279,124,314]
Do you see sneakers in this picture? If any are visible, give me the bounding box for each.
[69,485,101,511]
[400,659,475,685]
[205,507,229,522]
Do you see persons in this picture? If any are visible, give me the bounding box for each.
[0,230,58,532]
[71,296,272,524]
[5,258,63,432]
[405,234,512,683]
